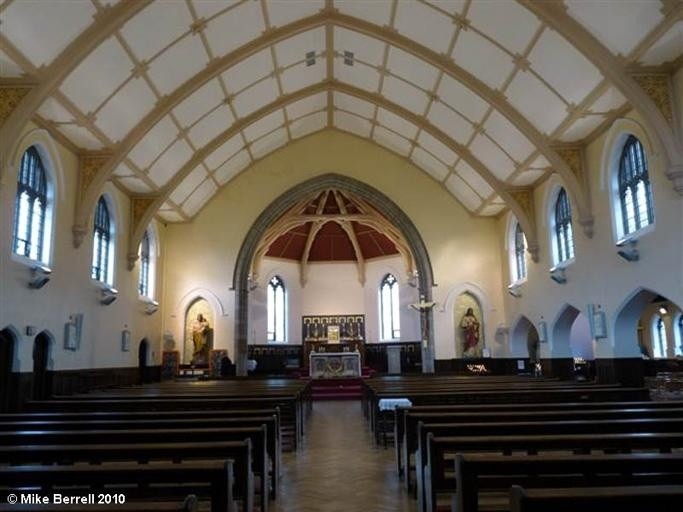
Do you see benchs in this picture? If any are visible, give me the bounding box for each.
[2,376,315,511]
[356,362,683,510]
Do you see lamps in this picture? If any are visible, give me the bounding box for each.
[100,289,117,305]
[612,243,643,266]
[27,267,51,289]
[550,270,567,284]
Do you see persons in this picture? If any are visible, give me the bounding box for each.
[304,317,363,338]
[192,314,211,362]
[459,307,480,354]
[218,350,233,378]
